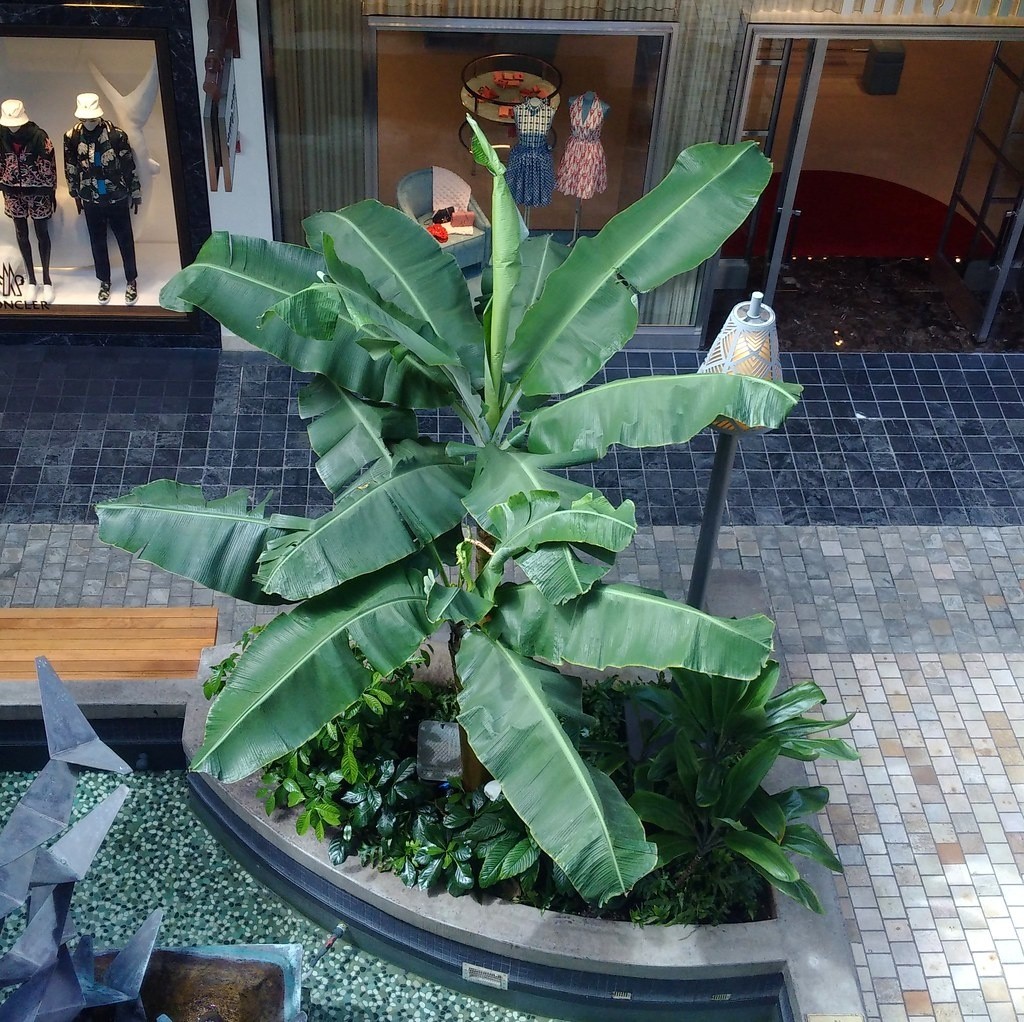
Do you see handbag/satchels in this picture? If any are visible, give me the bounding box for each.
[451,210,474,227]
[424,206,454,225]
[427,224,448,243]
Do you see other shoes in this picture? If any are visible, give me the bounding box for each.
[25,282,39,304]
[42,281,55,304]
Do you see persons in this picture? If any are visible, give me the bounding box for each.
[0,98,57,304]
[554,91,611,199]
[506,97,556,208]
[62,93,142,305]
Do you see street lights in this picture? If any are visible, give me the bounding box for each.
[690,290,782,612]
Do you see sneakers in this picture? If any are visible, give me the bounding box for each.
[98,280,112,304]
[125,280,138,306]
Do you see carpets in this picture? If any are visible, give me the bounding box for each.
[718,170,994,258]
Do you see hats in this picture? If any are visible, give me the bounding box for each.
[74,93,104,119]
[0,99,29,127]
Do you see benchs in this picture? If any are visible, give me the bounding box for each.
[0,607,220,680]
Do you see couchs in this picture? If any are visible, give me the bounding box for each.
[396,165,491,271]
